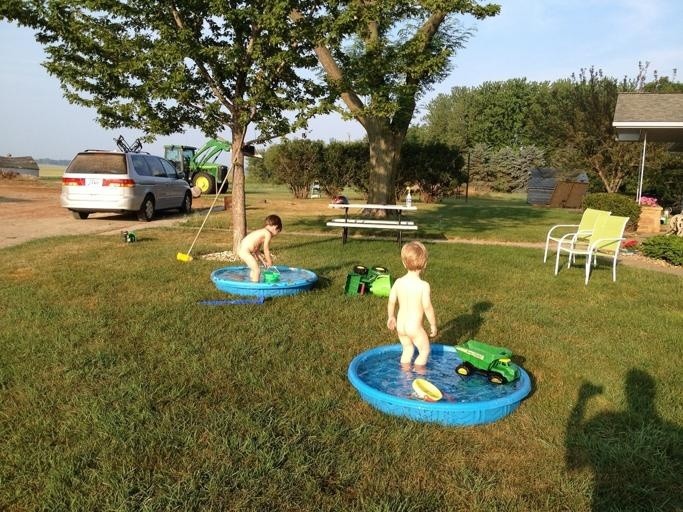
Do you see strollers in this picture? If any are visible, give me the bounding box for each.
[311,180,321,199]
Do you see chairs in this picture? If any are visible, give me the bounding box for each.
[554,214,631,285]
[543,208,612,269]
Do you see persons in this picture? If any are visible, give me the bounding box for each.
[236,214,282,282]
[386,239,437,367]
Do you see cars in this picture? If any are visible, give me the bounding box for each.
[60,135,192,222]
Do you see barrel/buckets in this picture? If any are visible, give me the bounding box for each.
[263,266,279,284]
[411,378,443,401]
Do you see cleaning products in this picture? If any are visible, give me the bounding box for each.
[406,189,412,208]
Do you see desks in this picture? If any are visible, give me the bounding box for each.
[329,203,417,248]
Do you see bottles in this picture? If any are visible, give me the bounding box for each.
[406,191,413,209]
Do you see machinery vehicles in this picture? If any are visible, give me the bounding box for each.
[164,136,264,194]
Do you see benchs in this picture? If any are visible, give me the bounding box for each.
[332,219,415,243]
[325,222,418,247]
